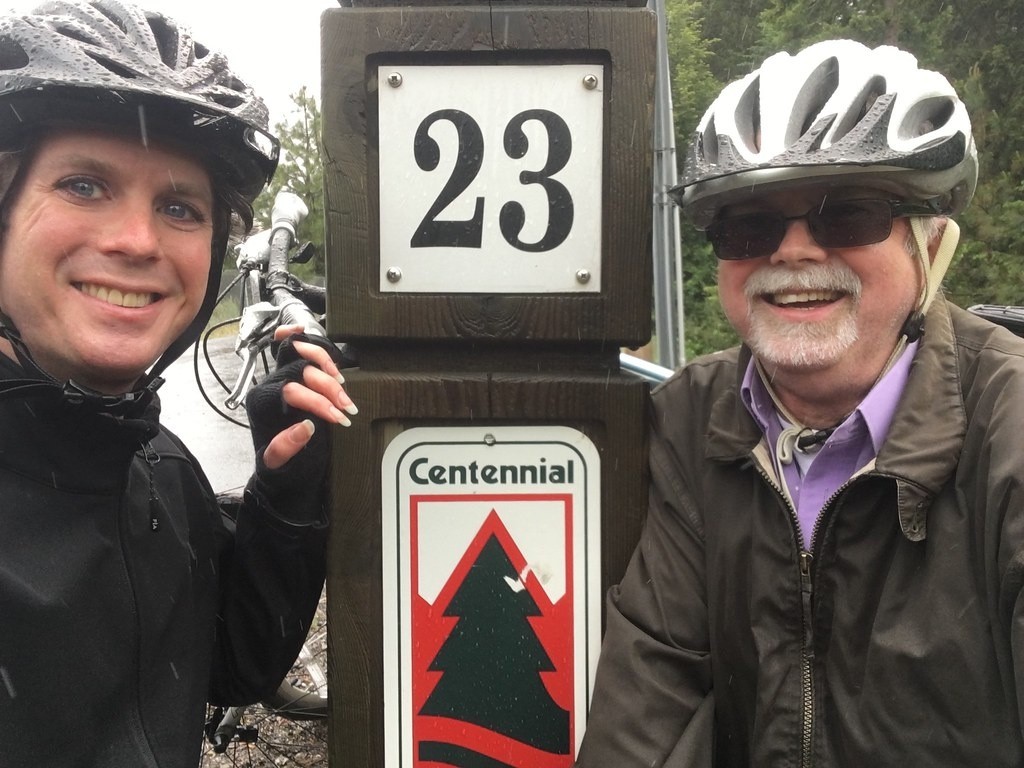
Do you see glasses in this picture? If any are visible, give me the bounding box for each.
[704,198,938,260]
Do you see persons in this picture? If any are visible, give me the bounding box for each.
[573,38,1024,768]
[0,0,356,768]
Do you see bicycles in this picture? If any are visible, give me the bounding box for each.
[187,190,1023,765]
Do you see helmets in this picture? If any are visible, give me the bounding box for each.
[0,0,281,202]
[667,40,978,221]
